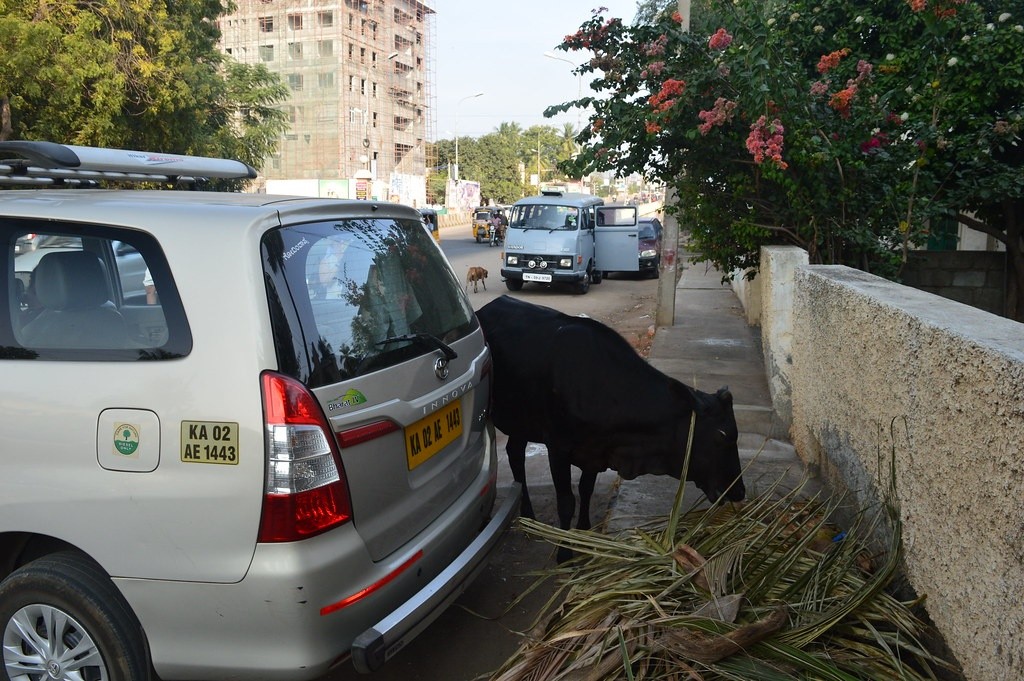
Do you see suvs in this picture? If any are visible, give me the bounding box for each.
[0,141,523,681]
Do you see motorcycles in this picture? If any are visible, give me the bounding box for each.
[634,199,637,205]
[472,206,508,243]
[625,200,630,205]
[417,208,440,246]
[613,198,616,204]
[487,222,501,248]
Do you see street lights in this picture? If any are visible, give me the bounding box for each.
[455,92,483,180]
[366,52,398,173]
[542,53,581,155]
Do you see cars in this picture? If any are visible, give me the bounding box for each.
[618,218,663,280]
[648,194,657,203]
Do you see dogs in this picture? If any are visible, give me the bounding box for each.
[465,266,488,293]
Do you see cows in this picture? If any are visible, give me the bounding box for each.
[476,294,746,565]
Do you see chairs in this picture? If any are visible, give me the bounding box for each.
[13,251,136,350]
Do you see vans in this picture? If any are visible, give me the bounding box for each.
[498,191,642,295]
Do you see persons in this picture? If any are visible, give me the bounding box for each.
[487,210,503,239]
[20,266,61,339]
[612,193,638,205]
[143,268,158,305]
